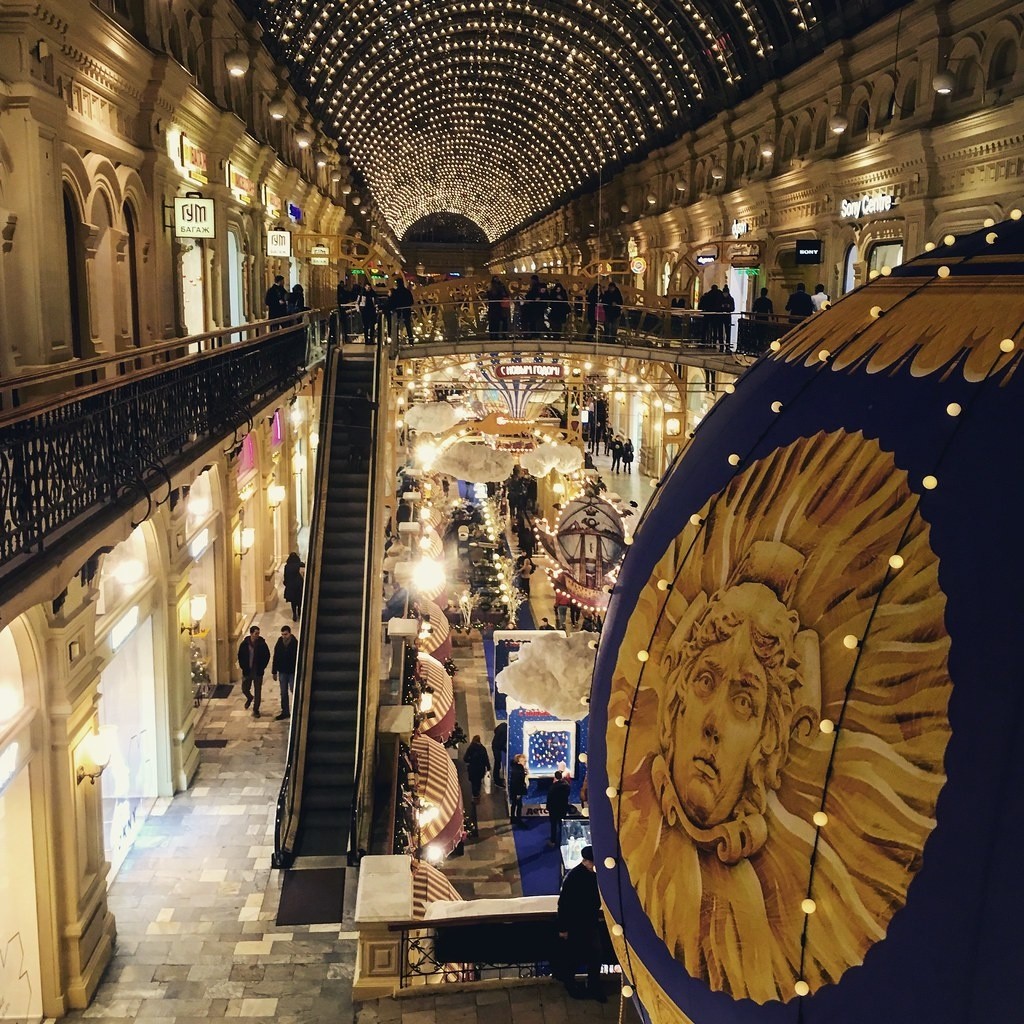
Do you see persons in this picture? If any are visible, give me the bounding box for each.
[238,626,271,719]
[604,282,623,343]
[556,845,611,1005]
[610,435,634,474]
[785,282,815,323]
[752,287,775,321]
[698,284,735,352]
[561,385,608,441]
[288,284,305,334]
[539,587,602,632]
[265,275,291,332]
[284,552,305,622]
[589,421,614,456]
[422,276,468,313]
[811,284,831,313]
[384,384,538,598]
[485,275,572,340]
[338,278,414,346]
[272,625,298,720]
[661,295,692,319]
[574,283,604,341]
[464,722,570,847]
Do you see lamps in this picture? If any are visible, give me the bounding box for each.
[620,203,630,213]
[315,145,330,166]
[341,178,352,194]
[932,55,968,95]
[366,216,388,238]
[711,158,742,180]
[267,484,286,511]
[351,190,361,206]
[359,204,367,216]
[195,33,249,75]
[828,104,870,142]
[253,88,290,120]
[330,165,341,182]
[294,121,316,149]
[234,528,253,557]
[677,172,703,191]
[512,225,582,255]
[647,188,669,203]
[181,594,206,634]
[760,132,794,158]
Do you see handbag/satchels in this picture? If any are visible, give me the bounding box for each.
[501,297,510,307]
[595,305,605,321]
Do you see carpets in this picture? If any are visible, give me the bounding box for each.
[212,684,235,699]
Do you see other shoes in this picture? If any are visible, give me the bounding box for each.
[245,695,254,708]
[253,708,260,718]
[276,712,291,720]
[495,783,505,788]
[587,986,606,1004]
[293,617,296,621]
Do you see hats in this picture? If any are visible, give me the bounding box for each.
[581,846,593,860]
